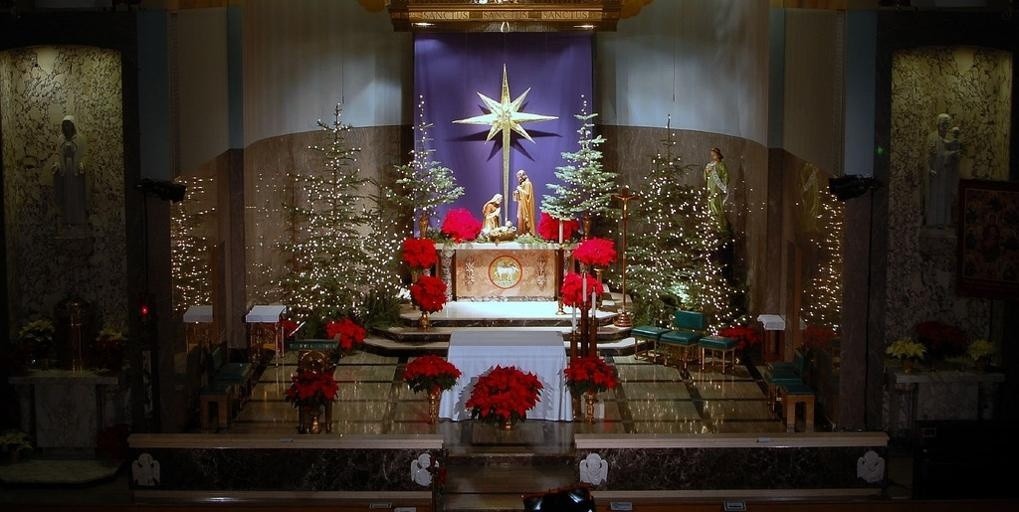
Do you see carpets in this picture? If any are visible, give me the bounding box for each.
[1,459,118,490]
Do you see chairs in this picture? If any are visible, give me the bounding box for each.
[209,346,253,408]
[658,310,704,370]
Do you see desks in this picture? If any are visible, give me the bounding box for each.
[443,329,566,421]
[245,304,288,368]
[182,303,214,376]
[892,369,1006,438]
[8,369,121,459]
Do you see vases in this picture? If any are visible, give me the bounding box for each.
[978,357,989,369]
[899,356,914,372]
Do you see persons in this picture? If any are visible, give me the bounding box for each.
[979,209,1003,261]
[479,193,503,235]
[511,168,535,236]
[701,147,731,234]
[923,112,961,228]
[940,126,960,169]
[47,113,89,227]
[489,221,513,233]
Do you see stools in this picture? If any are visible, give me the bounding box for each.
[764,347,815,434]
[631,325,672,363]
[695,335,738,373]
[199,381,233,431]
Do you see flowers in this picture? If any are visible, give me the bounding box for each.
[16,316,56,343]
[283,369,340,406]
[94,316,129,346]
[401,206,484,315]
[326,317,369,354]
[966,337,998,362]
[539,211,623,398]
[883,335,926,363]
[401,355,545,426]
